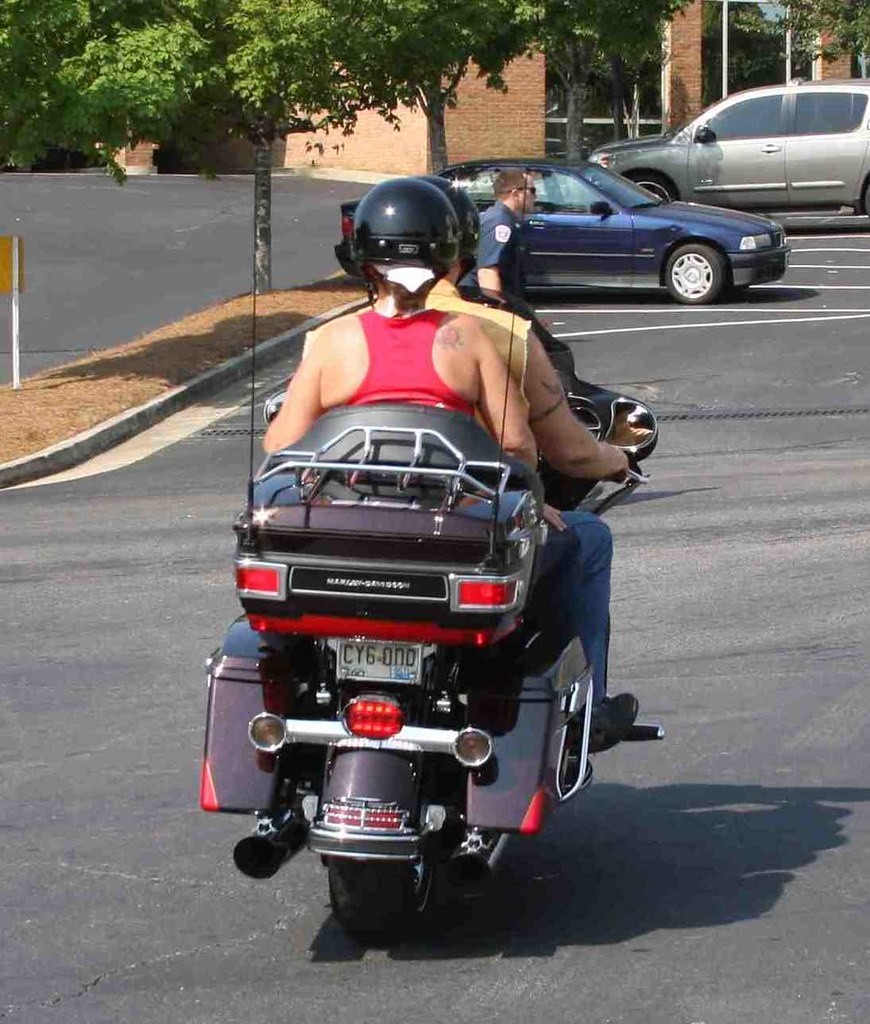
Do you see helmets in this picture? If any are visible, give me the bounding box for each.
[413,176,480,273]
[351,178,460,280]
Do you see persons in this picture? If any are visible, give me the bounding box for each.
[603,403,650,445]
[263,176,639,753]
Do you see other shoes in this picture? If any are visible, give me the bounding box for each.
[569,693,638,756]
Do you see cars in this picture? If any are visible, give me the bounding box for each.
[332,156,792,307]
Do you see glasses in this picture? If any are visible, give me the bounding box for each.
[508,187,535,196]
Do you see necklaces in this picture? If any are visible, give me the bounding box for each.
[460,167,551,329]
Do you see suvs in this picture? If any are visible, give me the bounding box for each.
[588,76,870,219]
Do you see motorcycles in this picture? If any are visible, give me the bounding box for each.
[196,372,658,941]
